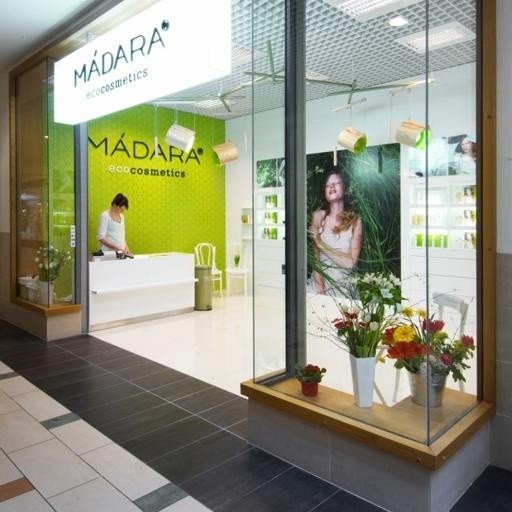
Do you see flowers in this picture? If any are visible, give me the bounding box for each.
[35,245,71,281]
[383,306,475,381]
[296,364,326,382]
[306,272,407,357]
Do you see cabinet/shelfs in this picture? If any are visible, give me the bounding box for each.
[407,182,476,254]
[241,189,286,242]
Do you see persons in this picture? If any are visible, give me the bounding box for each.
[264,197,272,237]
[97,193,129,254]
[448,135,476,175]
[307,170,362,301]
[463,187,474,247]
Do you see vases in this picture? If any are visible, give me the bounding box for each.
[350,354,375,409]
[407,371,446,406]
[17,277,54,305]
[302,382,317,396]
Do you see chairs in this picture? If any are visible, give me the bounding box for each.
[225,243,249,298]
[195,243,223,298]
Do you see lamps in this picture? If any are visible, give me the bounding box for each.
[153,41,436,166]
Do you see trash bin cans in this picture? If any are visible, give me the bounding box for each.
[195,266,212,311]
